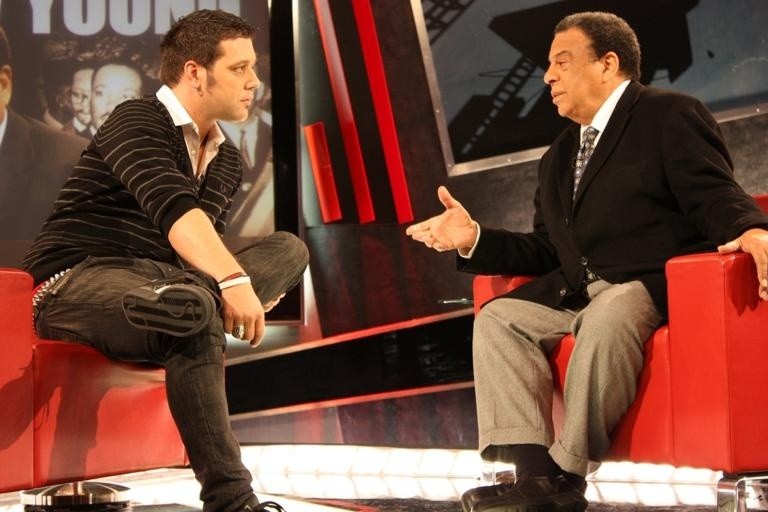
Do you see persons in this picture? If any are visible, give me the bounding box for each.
[16,6,311,512]
[0,25,275,265]
[403,9,768,511]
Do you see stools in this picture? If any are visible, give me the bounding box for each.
[0,261,192,511]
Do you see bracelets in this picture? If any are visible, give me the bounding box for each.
[218,271,250,283]
[218,275,254,290]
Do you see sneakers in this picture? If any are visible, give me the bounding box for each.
[234,494,286,512]
[121,280,216,340]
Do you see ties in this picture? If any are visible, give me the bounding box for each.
[571,127,599,205]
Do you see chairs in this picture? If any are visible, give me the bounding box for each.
[466,187,768,512]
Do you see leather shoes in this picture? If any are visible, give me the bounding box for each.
[460,474,587,512]
[473,468,589,512]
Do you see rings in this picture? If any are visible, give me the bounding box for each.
[231,326,247,340]
